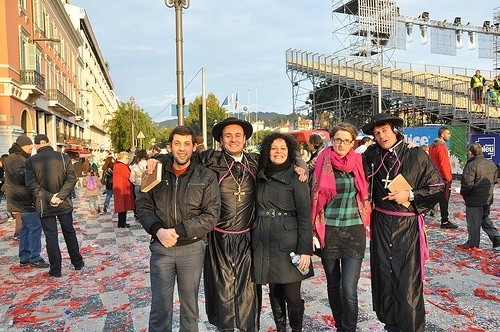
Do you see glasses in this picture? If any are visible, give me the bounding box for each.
[333,138,352,145]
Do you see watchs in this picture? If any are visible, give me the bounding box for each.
[409,189,415,201]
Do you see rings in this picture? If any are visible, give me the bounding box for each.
[304,171,308,176]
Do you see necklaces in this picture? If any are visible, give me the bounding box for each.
[222,150,247,202]
[379,144,404,189]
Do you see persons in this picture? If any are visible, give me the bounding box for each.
[318,109,335,130]
[55,136,207,215]
[0,146,24,242]
[470,70,500,108]
[420,126,459,229]
[25,134,85,277]
[309,113,446,332]
[113,151,135,228]
[251,132,315,332]
[135,126,221,332]
[309,122,419,332]
[129,150,151,228]
[146,117,307,332]
[5,134,50,268]
[255,133,377,198]
[457,144,500,250]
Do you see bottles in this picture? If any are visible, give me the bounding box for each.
[290,251,310,275]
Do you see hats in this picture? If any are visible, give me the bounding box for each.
[212,117,253,141]
[16,136,32,147]
[34,134,49,145]
[362,113,403,135]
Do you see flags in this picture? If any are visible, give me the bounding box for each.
[234,91,238,109]
[220,94,228,109]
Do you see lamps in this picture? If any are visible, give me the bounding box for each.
[33,38,60,42]
[97,104,104,106]
[76,89,92,93]
[104,113,111,115]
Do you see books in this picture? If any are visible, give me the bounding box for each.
[386,174,416,209]
[140,163,162,193]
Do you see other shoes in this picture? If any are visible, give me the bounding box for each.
[440,221,458,228]
[457,242,479,249]
[75,262,84,269]
[49,270,61,277]
[493,237,500,248]
[28,258,49,267]
[20,259,29,266]
[118,223,130,228]
[14,234,19,240]
[103,207,107,212]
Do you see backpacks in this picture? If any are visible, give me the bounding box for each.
[87,176,97,191]
